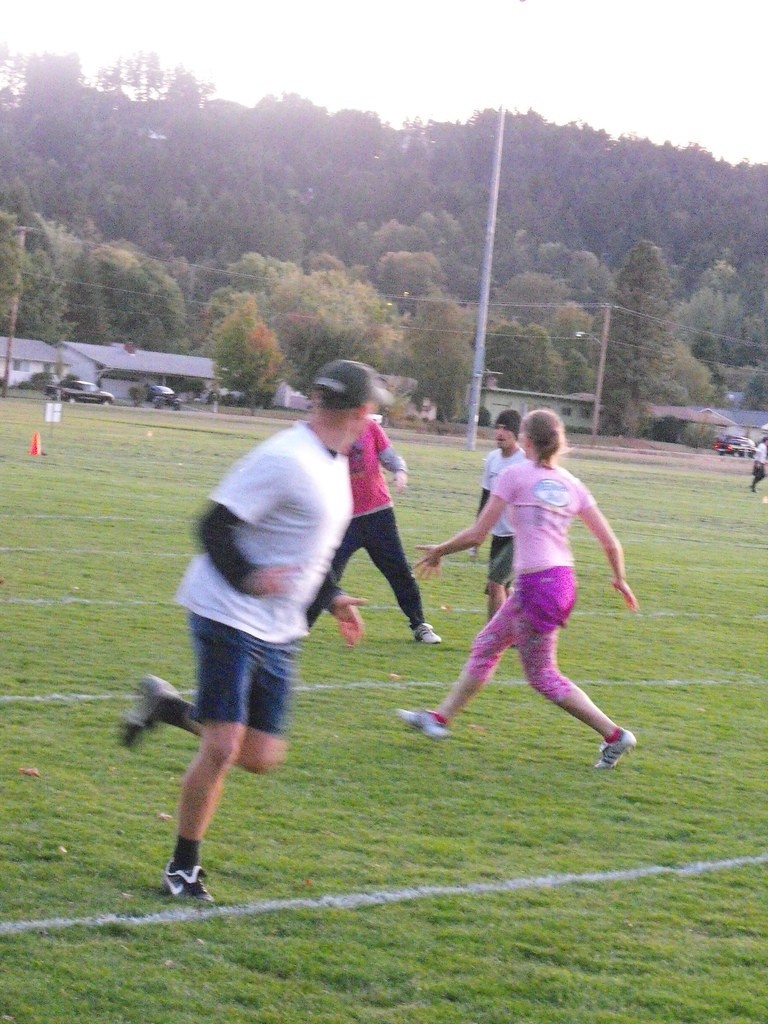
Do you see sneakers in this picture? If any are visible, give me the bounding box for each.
[395,708,448,741]
[161,862,214,903]
[596,729,636,770]
[120,672,167,748]
[414,622,442,643]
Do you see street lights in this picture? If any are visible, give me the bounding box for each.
[574,329,609,449]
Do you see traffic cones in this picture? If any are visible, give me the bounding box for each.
[28,431,42,456]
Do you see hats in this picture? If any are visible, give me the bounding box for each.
[496,408,521,435]
[314,360,397,410]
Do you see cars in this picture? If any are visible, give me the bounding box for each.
[712,433,757,457]
[144,383,182,409]
[44,378,115,406]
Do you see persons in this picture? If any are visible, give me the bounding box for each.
[312,419,442,643]
[121,360,392,904]
[470,410,526,649]
[397,410,640,767]
[750,437,768,491]
